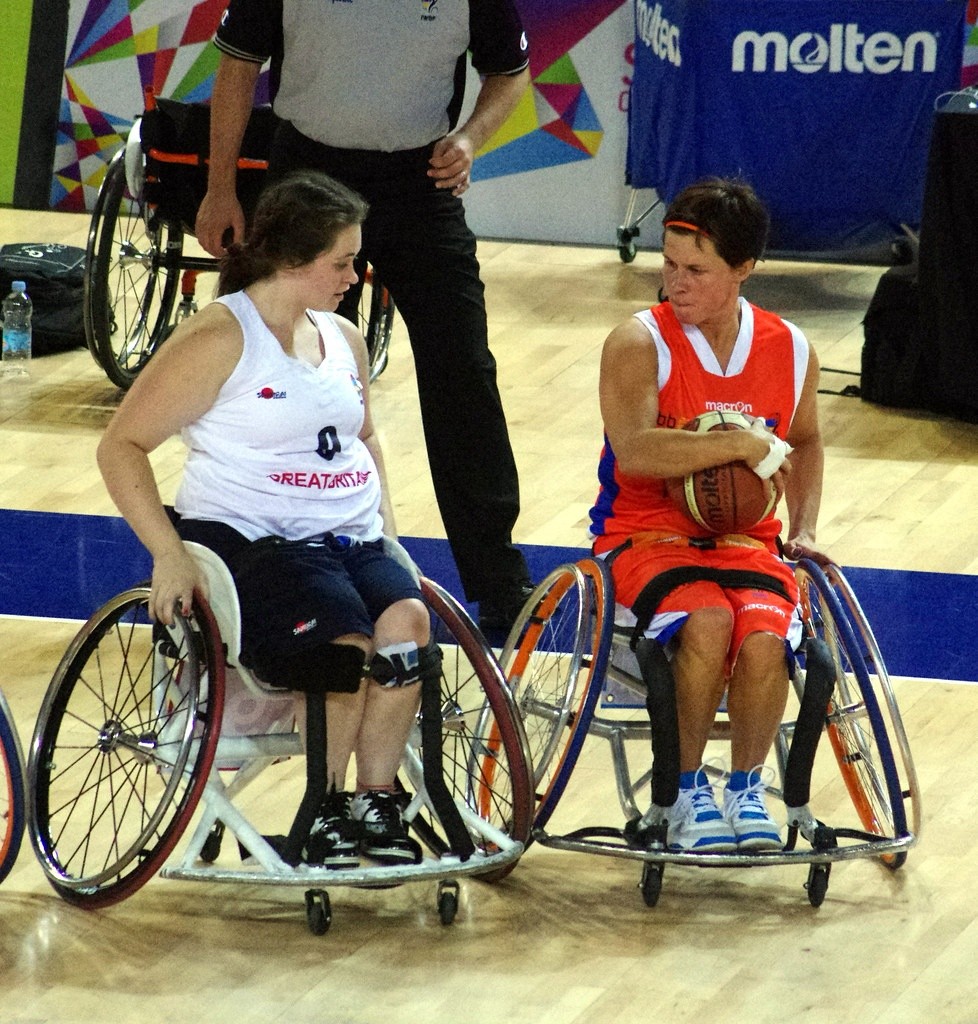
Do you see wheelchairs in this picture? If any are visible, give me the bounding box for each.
[466,557,920,909]
[26,504,535,937]
[84,96,395,393]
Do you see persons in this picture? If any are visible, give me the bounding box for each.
[597,181,825,856]
[94,174,433,870]
[196,1,564,627]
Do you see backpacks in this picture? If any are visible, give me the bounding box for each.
[0,243,118,350]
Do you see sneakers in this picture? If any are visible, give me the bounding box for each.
[665,757,738,852]
[479,581,562,623]
[300,772,360,870]
[723,765,784,852]
[350,787,416,861]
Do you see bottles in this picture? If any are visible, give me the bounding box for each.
[2,282,33,384]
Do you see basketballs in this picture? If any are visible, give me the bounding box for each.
[666,409,779,533]
